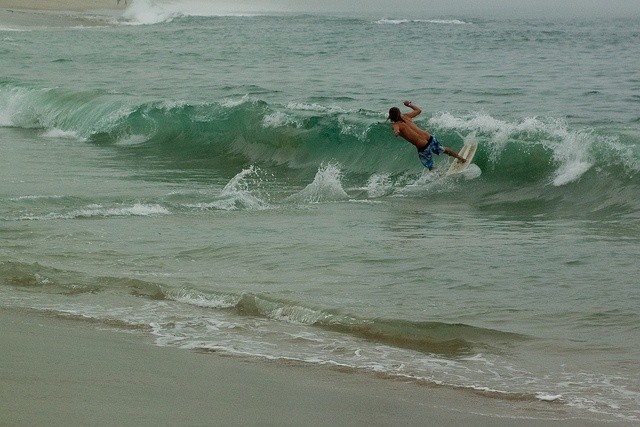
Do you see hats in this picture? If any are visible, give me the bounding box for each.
[387,107,401,122]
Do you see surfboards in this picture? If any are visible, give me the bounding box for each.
[447,141,478,173]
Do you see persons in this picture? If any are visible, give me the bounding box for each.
[388,98,467,171]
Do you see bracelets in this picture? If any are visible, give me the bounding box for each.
[408,101,412,107]
